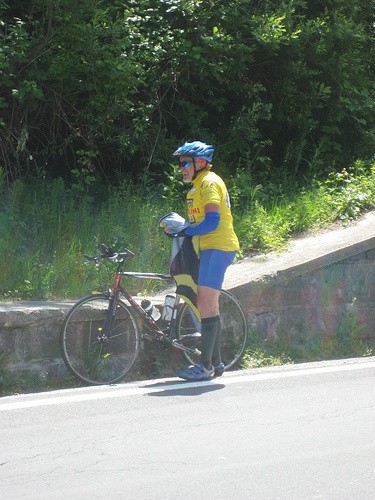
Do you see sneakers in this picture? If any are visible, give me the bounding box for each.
[177,360,215,379]
[215,362,225,376]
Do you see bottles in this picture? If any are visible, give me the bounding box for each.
[141,299,161,321]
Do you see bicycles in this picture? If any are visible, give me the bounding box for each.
[59,243,248,385]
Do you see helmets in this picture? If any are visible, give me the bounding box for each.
[173,141,215,162]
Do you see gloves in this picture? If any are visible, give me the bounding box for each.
[165,230,185,237]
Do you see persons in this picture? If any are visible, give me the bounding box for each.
[163,141,240,381]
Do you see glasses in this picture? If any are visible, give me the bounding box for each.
[179,161,192,168]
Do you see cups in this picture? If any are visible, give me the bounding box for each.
[162,295,175,321]
